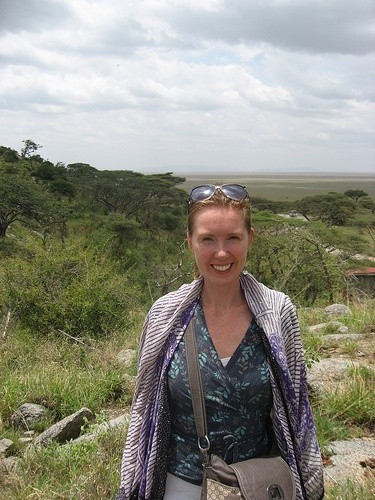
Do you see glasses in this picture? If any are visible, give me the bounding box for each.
[187,184,250,204]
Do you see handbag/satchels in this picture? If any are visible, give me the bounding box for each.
[200,454,296,500]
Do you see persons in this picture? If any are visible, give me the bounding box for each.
[121,184,325,499]
